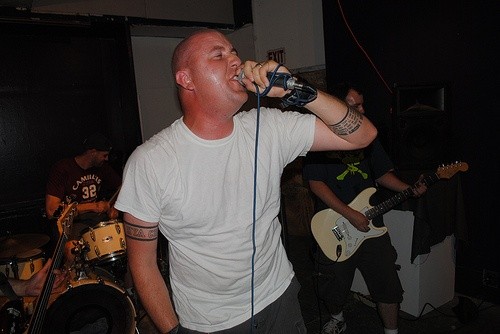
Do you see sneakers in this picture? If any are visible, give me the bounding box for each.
[321,318,347,334]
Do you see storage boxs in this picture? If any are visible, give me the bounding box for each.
[349,206,457,317]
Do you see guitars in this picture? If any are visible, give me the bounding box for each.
[310,161,470,264]
[23,204,79,332]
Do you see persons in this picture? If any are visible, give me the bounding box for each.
[302,82,426,334]
[32,132,122,243]
[115,29,378,334]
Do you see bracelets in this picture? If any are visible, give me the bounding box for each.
[281,76,318,112]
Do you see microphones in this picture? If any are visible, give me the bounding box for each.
[239,69,317,94]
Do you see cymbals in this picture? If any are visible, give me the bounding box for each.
[0,233,51,258]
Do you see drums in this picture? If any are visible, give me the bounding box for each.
[81,219,128,266]
[0,248,45,282]
[34,269,136,334]
